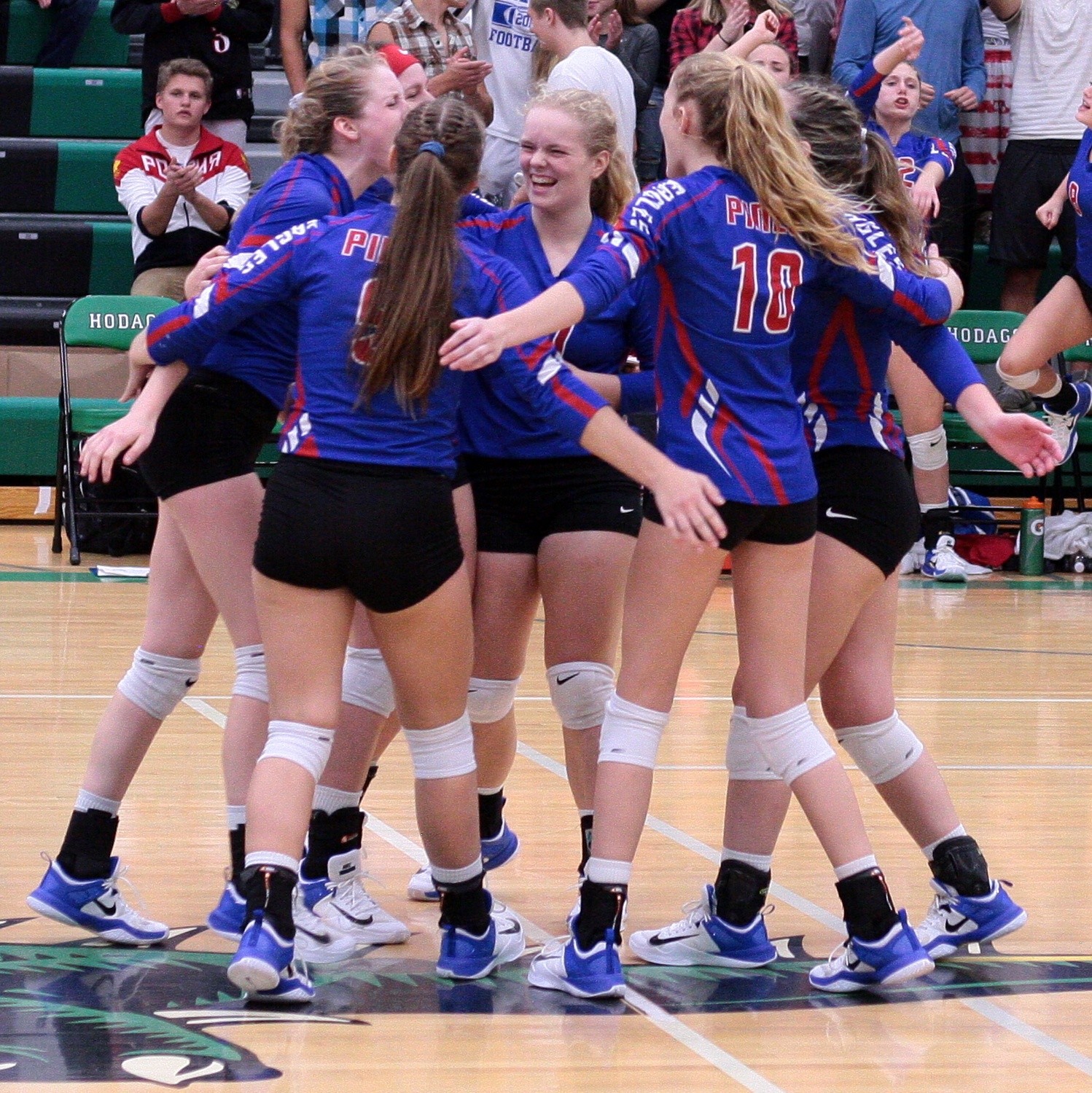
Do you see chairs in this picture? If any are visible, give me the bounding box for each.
[942,310,1062,517]
[1056,338,1092,513]
[51,295,181,564]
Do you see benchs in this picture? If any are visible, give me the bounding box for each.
[0,0,290,476]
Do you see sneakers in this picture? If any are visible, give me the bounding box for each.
[809,906,937,993]
[628,882,779,968]
[206,867,355,962]
[435,902,525,980]
[1043,380,1092,467]
[920,535,969,581]
[564,876,627,937]
[915,878,1027,959]
[408,820,519,901]
[227,909,316,1005]
[296,849,411,944]
[528,914,626,1000]
[26,850,170,945]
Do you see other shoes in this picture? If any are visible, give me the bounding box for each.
[992,381,1035,412]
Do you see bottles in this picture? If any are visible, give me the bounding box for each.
[1068,553,1086,572]
[1020,497,1044,577]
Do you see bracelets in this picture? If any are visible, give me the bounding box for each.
[717,31,732,46]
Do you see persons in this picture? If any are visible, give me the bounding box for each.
[33,0,1092,310]
[23,54,1066,1001]
[840,14,992,582]
[994,78,1092,463]
